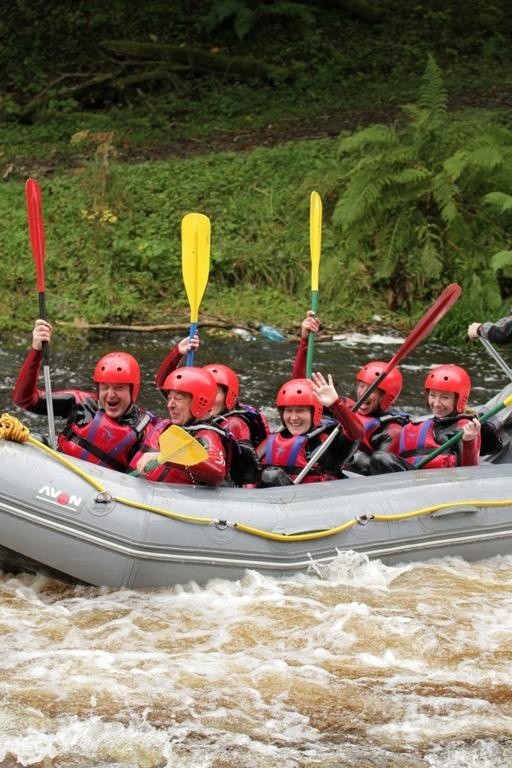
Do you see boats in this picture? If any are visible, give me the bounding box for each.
[0,427,511,589]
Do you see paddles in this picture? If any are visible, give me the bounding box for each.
[294,282,462,487]
[25,178,60,453]
[181,213,211,367]
[130,424,210,477]
[305,191,322,377]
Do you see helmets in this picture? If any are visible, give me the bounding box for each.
[91,351,140,403]
[423,364,471,415]
[277,378,324,429]
[354,361,404,413]
[162,366,218,418]
[202,362,240,410]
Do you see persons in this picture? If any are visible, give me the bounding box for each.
[346,364,482,476]
[155,334,271,452]
[121,366,230,486]
[467,306,512,346]
[293,310,410,456]
[14,319,159,471]
[242,371,364,490]
